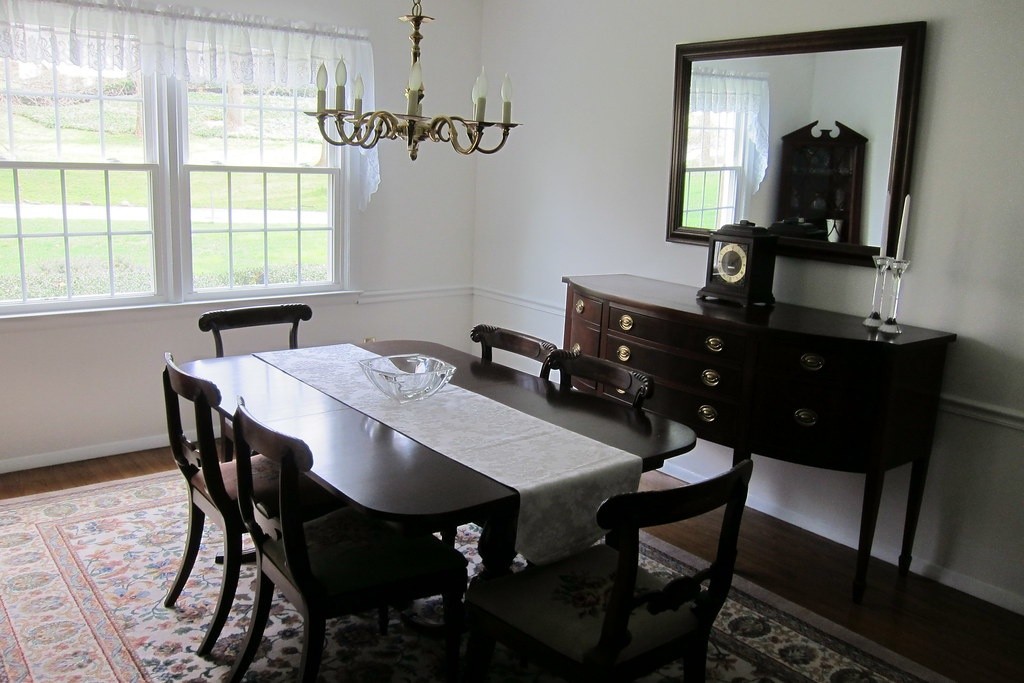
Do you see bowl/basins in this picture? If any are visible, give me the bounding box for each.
[358,353,456,404]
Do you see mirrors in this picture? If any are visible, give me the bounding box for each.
[666,21,928,271]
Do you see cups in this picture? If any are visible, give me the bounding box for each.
[826,219,844,242]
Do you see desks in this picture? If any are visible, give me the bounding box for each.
[182,341,697,683]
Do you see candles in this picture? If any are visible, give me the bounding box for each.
[880,190,888,257]
[895,194,911,261]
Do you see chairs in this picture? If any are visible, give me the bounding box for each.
[441,326,655,571]
[467,458,755,683]
[198,305,312,462]
[160,351,469,683]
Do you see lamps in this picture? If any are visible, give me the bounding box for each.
[304,0,522,163]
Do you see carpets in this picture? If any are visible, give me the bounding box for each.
[0,464,955,683]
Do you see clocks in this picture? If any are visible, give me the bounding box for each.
[696,219,778,307]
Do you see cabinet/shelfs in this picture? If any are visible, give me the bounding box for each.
[779,120,869,245]
[561,274,957,604]
